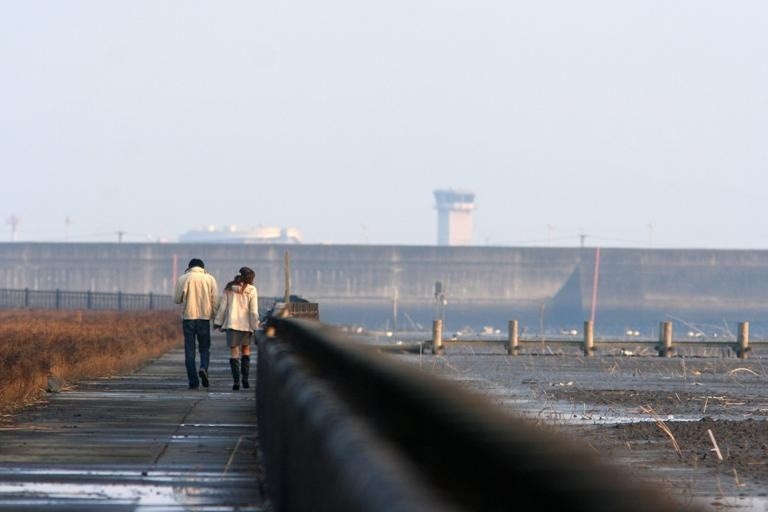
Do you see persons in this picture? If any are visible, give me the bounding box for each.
[173,258,220,388]
[213,266,261,390]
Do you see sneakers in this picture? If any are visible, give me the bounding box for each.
[199,369,209,387]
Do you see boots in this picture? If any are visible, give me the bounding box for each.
[241,355,250,387]
[230,357,240,390]
